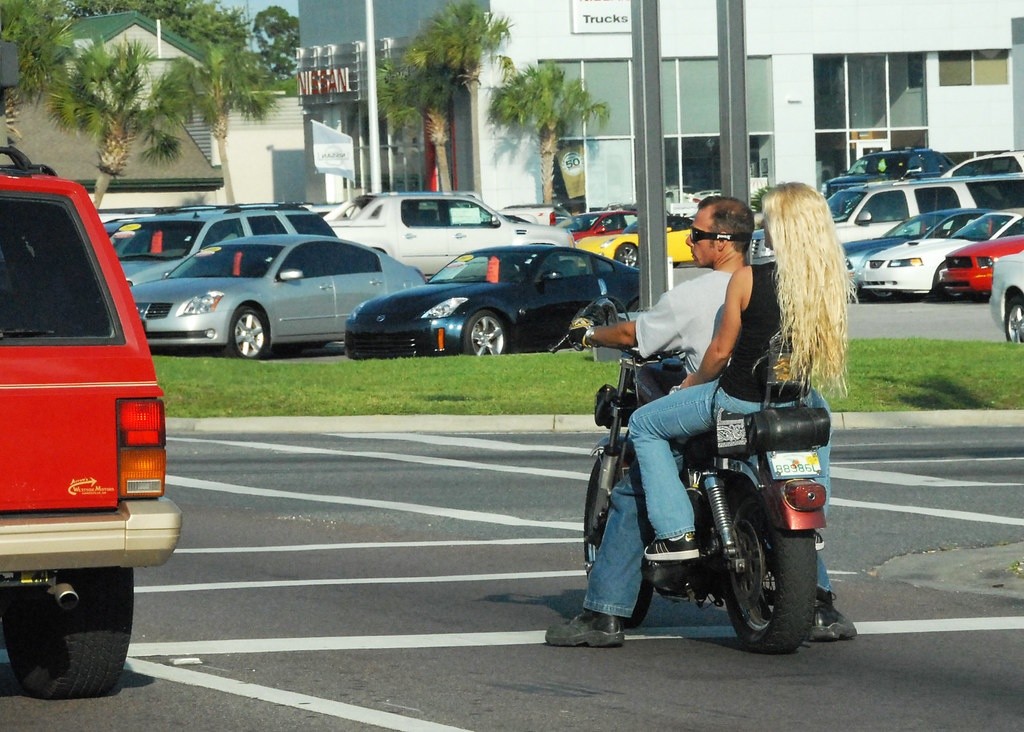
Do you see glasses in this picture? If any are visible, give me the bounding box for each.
[689,226,752,243]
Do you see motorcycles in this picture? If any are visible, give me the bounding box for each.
[553,297,830,655]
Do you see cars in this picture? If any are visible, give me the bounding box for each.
[945,234,1024,302]
[746,147,1023,279]
[1,145,182,701]
[500,183,723,270]
[129,234,429,359]
[344,243,640,360]
[860,208,1024,304]
[988,249,1024,345]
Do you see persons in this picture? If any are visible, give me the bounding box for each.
[543,183,858,649]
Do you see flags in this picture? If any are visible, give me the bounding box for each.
[310,118,356,189]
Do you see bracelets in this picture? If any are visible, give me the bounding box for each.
[584,326,602,348]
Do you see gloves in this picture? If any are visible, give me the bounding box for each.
[569,318,594,349]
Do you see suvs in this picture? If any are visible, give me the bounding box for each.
[324,191,576,282]
[101,202,341,289]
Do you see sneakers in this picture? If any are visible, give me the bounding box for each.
[808,599,857,641]
[815,532,824,549]
[545,609,624,647]
[644,530,699,560]
